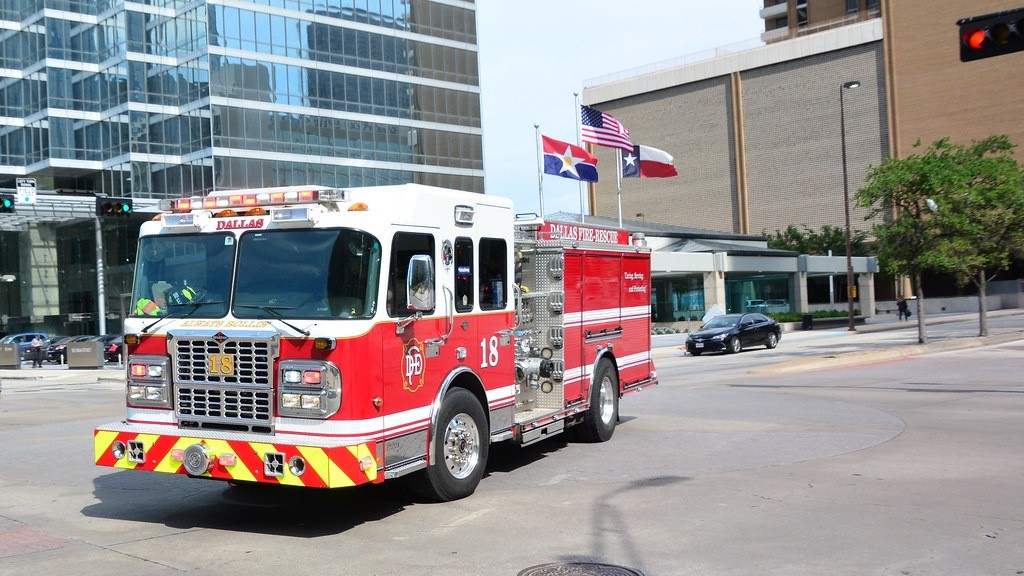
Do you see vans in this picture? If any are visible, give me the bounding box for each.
[0,332,50,356]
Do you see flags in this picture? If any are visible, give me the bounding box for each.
[579,104,634,152]
[620,144,678,179]
[540,133,598,184]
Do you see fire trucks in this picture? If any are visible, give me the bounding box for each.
[93,183,661,504]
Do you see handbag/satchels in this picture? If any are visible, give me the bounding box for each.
[906,310,911,316]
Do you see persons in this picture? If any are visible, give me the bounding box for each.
[31,335,43,368]
[896,295,908,320]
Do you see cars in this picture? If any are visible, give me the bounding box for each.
[685,312,783,356]
[31,333,123,364]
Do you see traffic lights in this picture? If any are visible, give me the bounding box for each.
[94,195,133,217]
[955,7,1023,63]
[0,194,16,213]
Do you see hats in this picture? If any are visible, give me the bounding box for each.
[36,335,40,337]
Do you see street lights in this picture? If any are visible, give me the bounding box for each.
[841,79,861,331]
